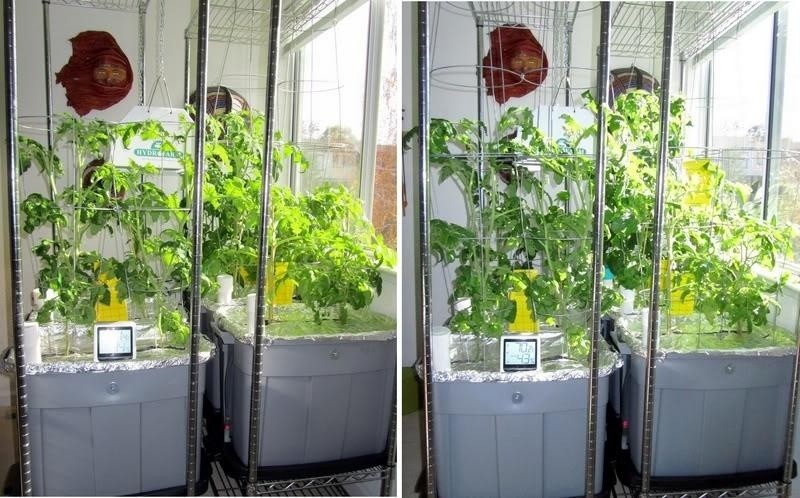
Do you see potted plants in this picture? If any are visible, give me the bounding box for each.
[20,103,798,497]
